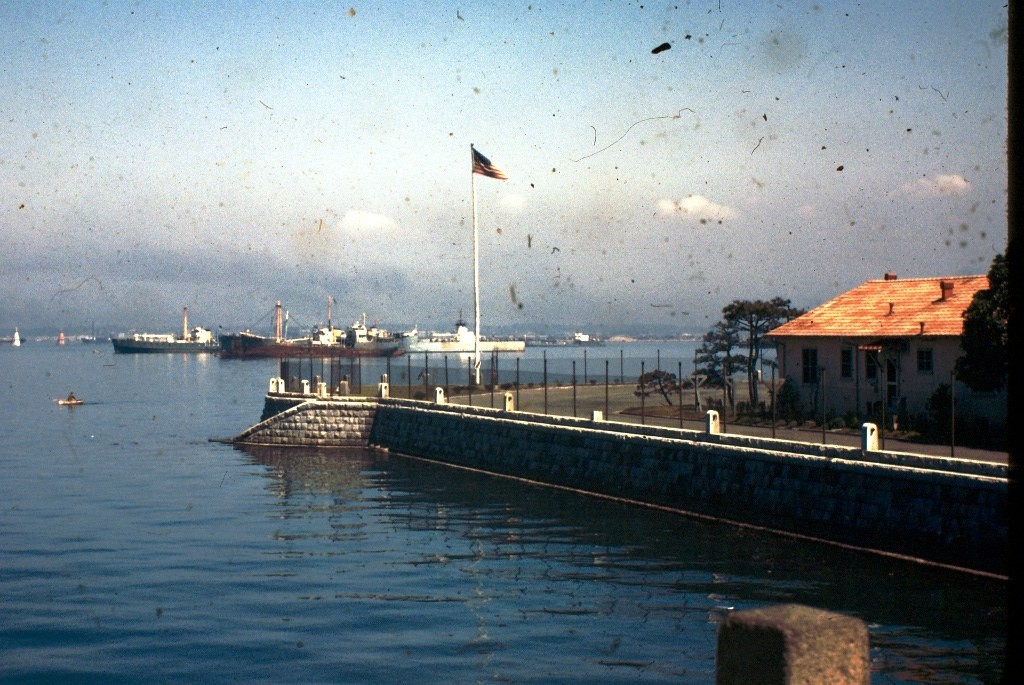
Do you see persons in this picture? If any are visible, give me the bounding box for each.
[68,392,77,401]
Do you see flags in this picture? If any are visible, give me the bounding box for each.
[472,148,507,179]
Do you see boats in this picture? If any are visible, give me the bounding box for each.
[399,324,524,352]
[215,299,407,358]
[109,307,219,353]
[51,398,85,405]
[10,326,20,348]
[564,332,606,348]
[0,333,26,344]
